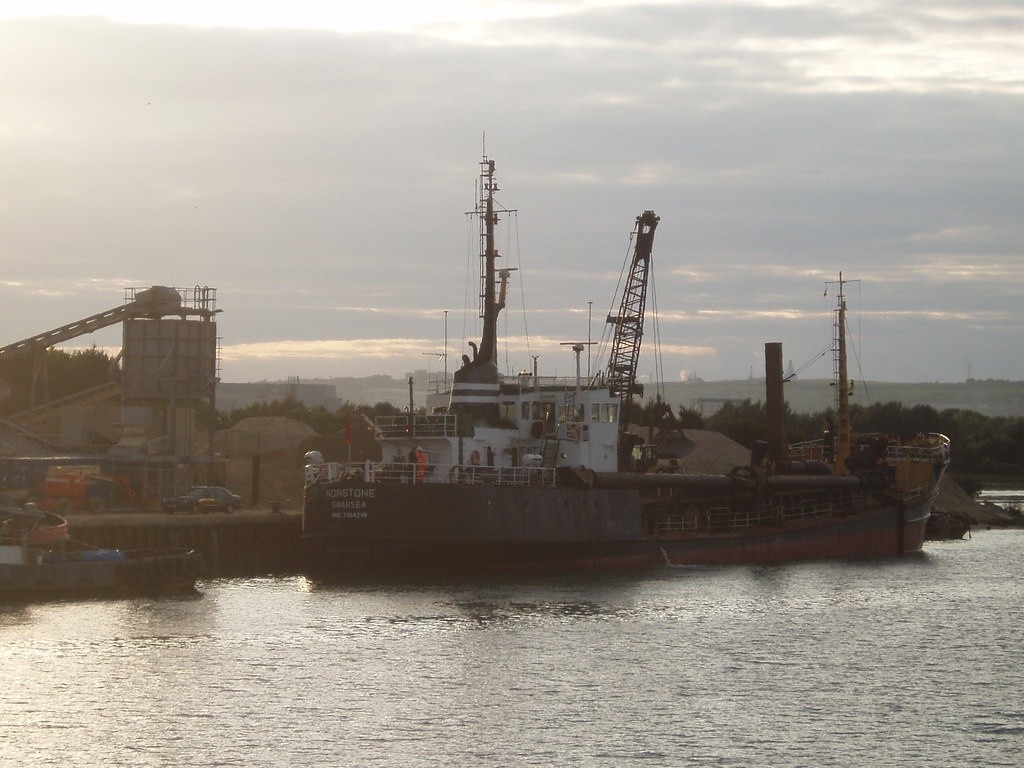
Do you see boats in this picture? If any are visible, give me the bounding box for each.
[297,135,950,562]
[1,503,199,596]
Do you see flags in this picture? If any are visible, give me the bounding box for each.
[343,411,352,448]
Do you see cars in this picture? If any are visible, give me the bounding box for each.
[164,486,244,515]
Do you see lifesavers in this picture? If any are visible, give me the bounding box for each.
[470,450,480,464]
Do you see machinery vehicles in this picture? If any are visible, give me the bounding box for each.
[568,208,698,473]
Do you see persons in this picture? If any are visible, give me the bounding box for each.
[407,447,426,483]
[487,447,495,486]
[393,448,406,480]
[471,450,480,485]
